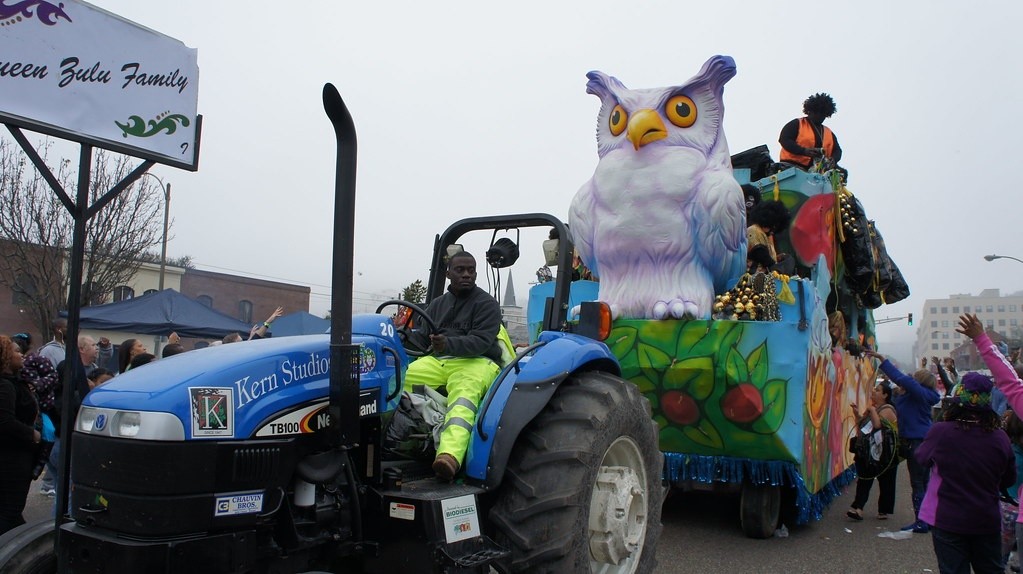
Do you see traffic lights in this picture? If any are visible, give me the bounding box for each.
[907,313,913,326]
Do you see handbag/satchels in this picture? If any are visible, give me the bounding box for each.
[917,461,943,525]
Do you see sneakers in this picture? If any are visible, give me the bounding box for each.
[41,487,56,498]
[433,453,458,483]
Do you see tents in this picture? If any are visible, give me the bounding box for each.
[59,288,273,340]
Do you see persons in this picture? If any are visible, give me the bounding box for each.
[779,92,842,171]
[914,371,1018,574]
[920,356,959,420]
[847,382,900,520]
[396,251,503,484]
[747,199,791,274]
[812,120,822,141]
[0,335,43,536]
[865,348,940,533]
[955,313,1023,574]
[827,297,847,347]
[38,306,283,518]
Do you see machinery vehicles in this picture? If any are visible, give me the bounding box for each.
[0,81,667,574]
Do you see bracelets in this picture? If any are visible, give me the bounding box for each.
[263,321,269,328]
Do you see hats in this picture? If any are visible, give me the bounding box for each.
[942,372,998,420]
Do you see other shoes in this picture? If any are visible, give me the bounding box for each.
[901,521,928,533]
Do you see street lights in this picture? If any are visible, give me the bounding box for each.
[140,172,173,359]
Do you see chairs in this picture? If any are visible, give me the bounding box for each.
[436,323,518,399]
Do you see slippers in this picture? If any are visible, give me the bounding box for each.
[878,512,887,520]
[847,510,863,520]
